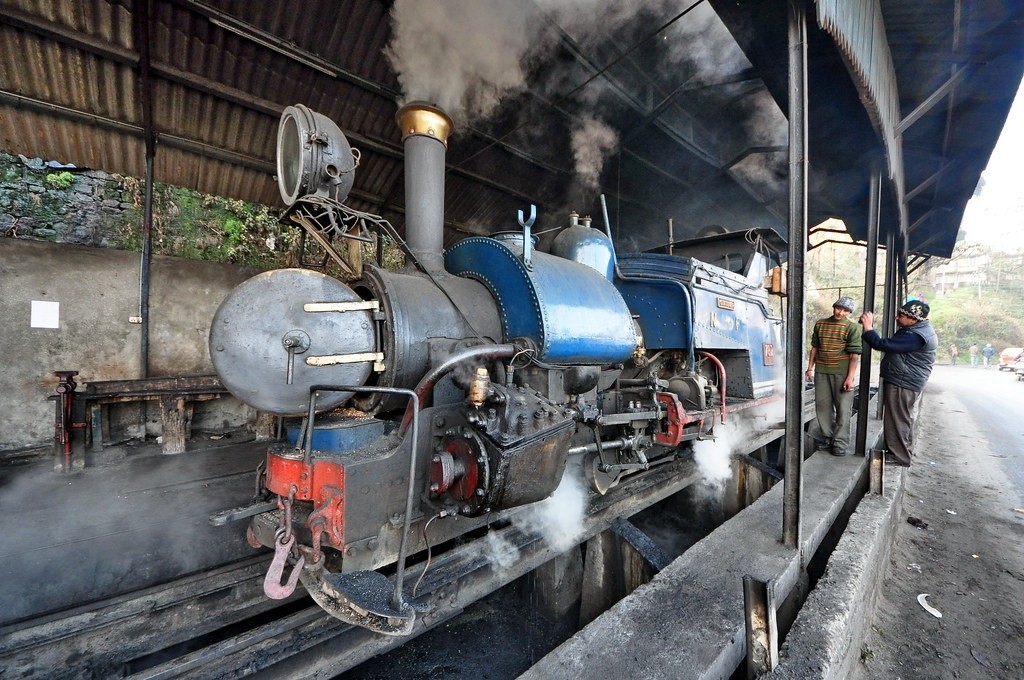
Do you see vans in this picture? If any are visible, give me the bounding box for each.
[997,347,1024,372]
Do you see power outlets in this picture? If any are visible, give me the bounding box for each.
[129,317,142,323]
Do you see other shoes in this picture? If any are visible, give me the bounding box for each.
[829,446,845,456]
[816,443,830,451]
[885,450,898,463]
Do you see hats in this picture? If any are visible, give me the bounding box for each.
[833,296,855,314]
[898,300,930,321]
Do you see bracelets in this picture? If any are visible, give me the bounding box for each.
[806,369,813,372]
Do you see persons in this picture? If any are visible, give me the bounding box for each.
[805,297,863,456]
[861,300,938,467]
[983,344,994,368]
[970,343,977,367]
[951,344,958,365]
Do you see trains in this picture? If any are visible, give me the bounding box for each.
[206,100,808,638]
